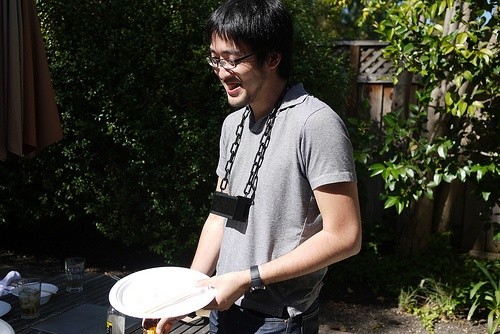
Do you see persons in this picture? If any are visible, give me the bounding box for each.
[142,0,362,334]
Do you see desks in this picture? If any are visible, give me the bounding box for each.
[0,271,210,334]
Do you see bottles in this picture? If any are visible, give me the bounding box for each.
[107,305,125,334]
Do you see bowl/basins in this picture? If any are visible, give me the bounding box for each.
[12,283,59,305]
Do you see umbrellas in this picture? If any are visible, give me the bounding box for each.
[0,0,66,162]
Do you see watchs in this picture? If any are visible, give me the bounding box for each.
[250,265,266,294]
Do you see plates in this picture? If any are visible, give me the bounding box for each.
[0,319,15,334]
[108,266,215,319]
[0,301,11,317]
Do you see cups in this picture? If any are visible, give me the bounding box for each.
[144,320,173,334]
[16,280,41,321]
[65,257,85,293]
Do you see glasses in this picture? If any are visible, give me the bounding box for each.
[206,52,256,70]
